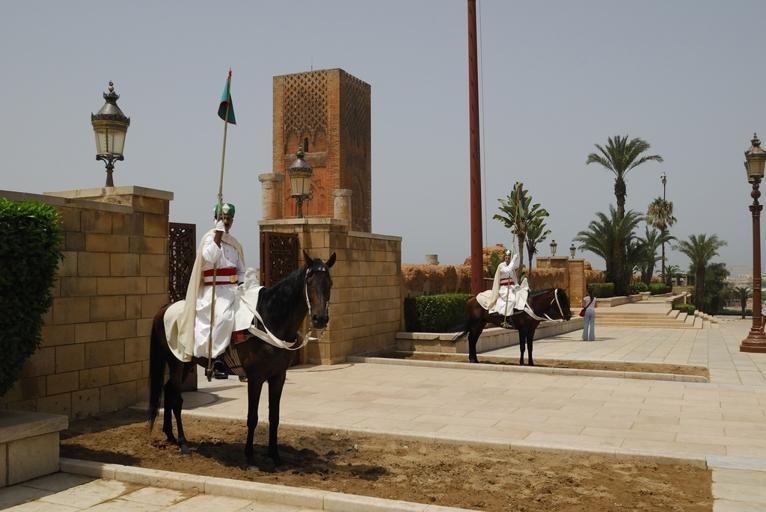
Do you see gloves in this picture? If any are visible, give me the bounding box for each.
[214,219,225,233]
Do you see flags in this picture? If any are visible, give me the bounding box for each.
[217,70,237,126]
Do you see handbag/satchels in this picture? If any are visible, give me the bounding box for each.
[579,308,586,317]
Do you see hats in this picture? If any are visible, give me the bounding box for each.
[215,203,236,217]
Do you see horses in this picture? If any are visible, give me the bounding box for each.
[451,287,572,366]
[147,248,338,474]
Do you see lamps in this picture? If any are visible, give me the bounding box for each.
[288,146,312,216]
[550,240,576,259]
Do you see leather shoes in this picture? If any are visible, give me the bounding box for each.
[204,363,227,380]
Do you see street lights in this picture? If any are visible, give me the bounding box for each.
[660,172,670,282]
[286,149,314,218]
[550,239,557,257]
[737,132,766,354]
[570,244,577,260]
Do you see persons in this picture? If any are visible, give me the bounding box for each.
[475,250,530,330]
[581,285,598,341]
[163,203,246,379]
